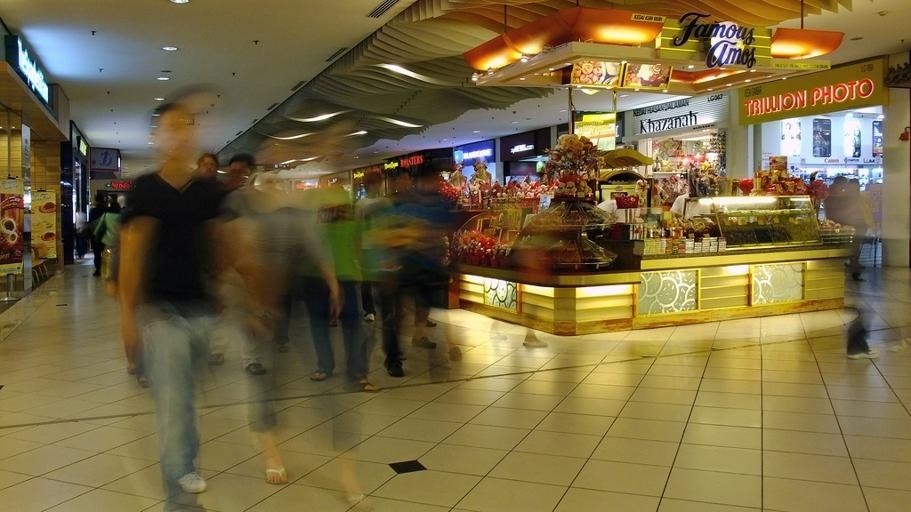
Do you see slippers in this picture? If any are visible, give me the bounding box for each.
[310,369,328,380]
[266,467,287,484]
[359,381,375,392]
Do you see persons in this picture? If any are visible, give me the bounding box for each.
[74,103,466,494]
[825,176,907,359]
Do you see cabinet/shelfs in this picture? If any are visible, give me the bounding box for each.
[668,193,825,251]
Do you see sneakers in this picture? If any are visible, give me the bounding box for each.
[207,353,224,364]
[329,320,337,326]
[413,336,435,348]
[388,366,405,377]
[363,312,374,321]
[179,471,207,494]
[128,362,151,387]
[427,320,436,327]
[249,364,264,375]
[279,345,288,352]
